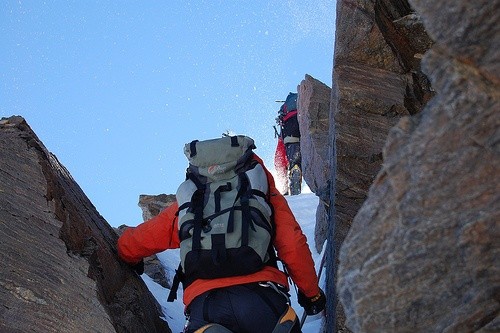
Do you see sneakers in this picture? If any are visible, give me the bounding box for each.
[290,170,301,195]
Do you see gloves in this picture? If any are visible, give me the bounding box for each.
[297,287,327,315]
[128,259,145,275]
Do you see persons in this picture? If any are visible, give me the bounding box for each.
[117,135,326,333]
[277,92,303,195]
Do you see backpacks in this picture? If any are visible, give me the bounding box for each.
[285,92,297,112]
[175,135,274,279]
